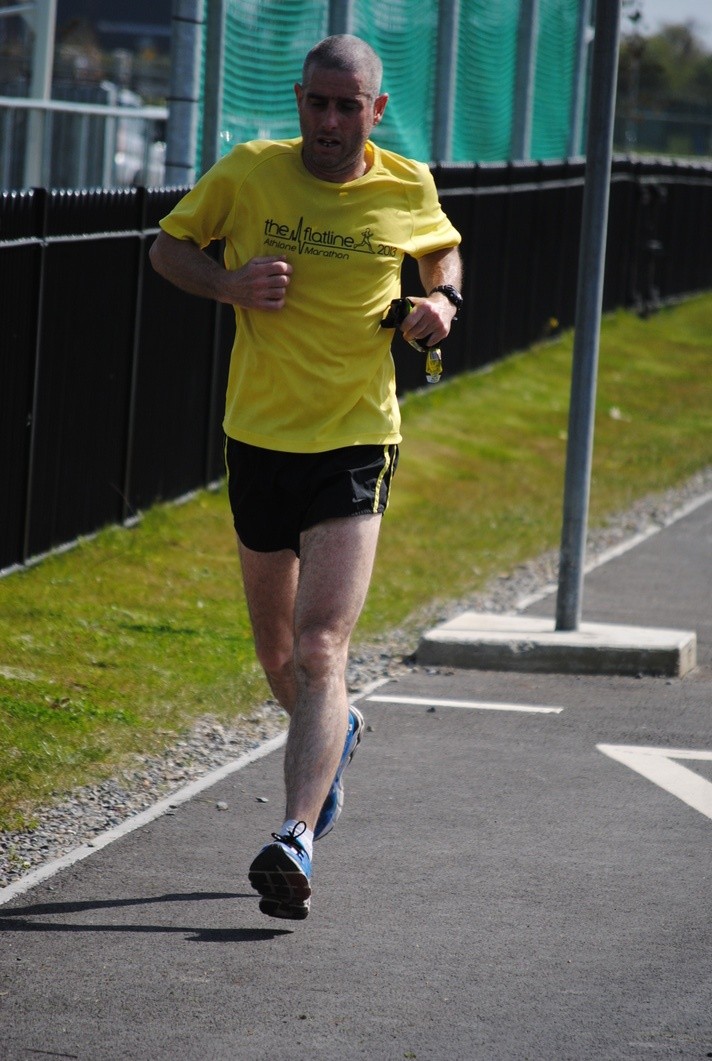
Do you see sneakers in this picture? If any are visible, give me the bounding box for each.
[311,704,366,841]
[248,820,310,920]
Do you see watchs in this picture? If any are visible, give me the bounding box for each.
[427,284,464,320]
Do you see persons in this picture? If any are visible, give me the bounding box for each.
[146,34,465,919]
